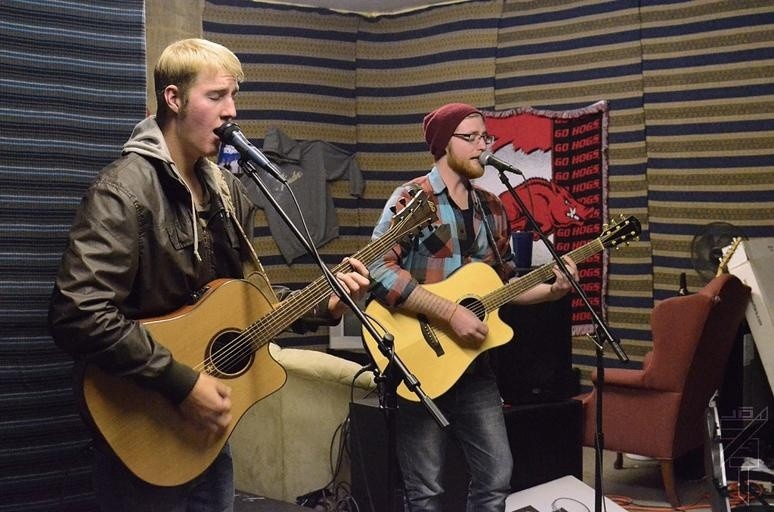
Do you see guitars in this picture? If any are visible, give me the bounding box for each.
[84,188,439,486]
[360,213,643,402]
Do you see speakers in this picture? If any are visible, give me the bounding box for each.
[348,289,583,512]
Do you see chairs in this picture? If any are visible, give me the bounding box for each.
[569,274,752,508]
[227,341,379,503]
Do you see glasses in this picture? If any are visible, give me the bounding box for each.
[451,132,497,148]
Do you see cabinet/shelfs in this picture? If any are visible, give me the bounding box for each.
[348,397,583,512]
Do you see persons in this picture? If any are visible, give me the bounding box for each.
[366,101,581,511]
[44,36,372,510]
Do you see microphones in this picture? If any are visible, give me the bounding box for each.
[479,151,523,175]
[218,122,288,185]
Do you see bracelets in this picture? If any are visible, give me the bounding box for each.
[448,304,459,325]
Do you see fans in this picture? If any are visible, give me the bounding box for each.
[690,221,748,284]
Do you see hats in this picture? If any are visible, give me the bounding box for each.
[422,101,487,163]
